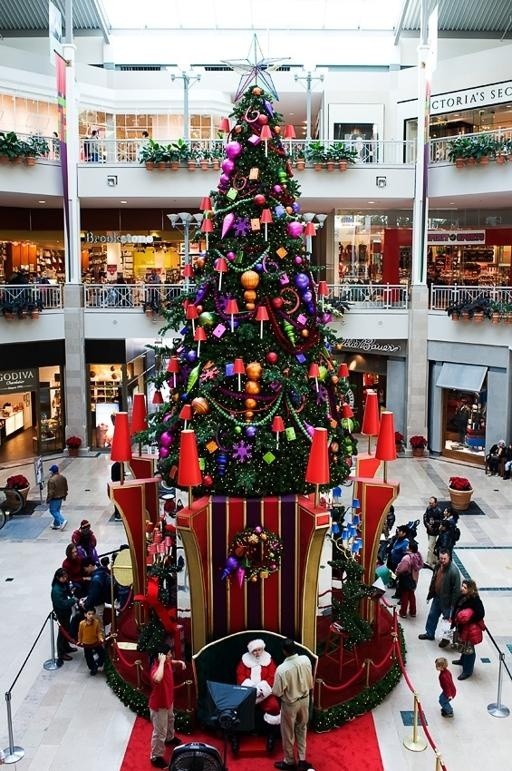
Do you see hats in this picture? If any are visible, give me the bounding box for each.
[80,520,91,529]
[245,638,266,653]
[49,464,59,473]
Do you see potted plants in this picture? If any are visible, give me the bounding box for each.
[447,133,512,169]
[141,302,154,319]
[0,131,51,167]
[443,295,512,327]
[2,299,44,323]
[287,139,362,172]
[137,137,220,171]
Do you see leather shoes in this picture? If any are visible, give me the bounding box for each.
[297,759,312,770]
[402,615,406,618]
[397,602,401,605]
[457,672,471,680]
[391,595,398,598]
[273,761,296,770]
[452,660,462,665]
[438,639,450,647]
[418,633,435,640]
[411,614,416,617]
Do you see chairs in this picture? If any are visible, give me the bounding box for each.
[485,452,506,479]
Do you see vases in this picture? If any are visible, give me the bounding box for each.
[396,443,403,452]
[447,487,474,512]
[68,447,80,457]
[411,447,425,457]
[2,485,31,508]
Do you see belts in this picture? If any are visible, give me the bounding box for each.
[294,694,310,702]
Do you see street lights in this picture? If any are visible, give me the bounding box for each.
[168,52,206,155]
[294,56,326,166]
[296,211,331,265]
[163,206,204,286]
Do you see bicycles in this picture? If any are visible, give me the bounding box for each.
[0,503,11,530]
[0,485,25,516]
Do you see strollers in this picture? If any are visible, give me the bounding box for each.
[378,517,423,560]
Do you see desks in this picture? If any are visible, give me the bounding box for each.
[32,436,57,452]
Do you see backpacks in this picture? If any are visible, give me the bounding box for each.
[93,571,117,604]
[453,526,461,541]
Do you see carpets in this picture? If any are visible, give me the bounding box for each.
[0,499,40,515]
[121,711,386,771]
[436,501,486,515]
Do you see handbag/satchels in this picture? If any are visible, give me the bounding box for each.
[398,571,415,593]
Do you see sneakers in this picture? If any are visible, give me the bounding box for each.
[90,668,98,676]
[49,523,61,529]
[59,655,73,660]
[60,520,68,530]
[66,647,77,653]
[441,708,454,718]
[150,756,168,768]
[165,735,181,745]
[115,517,122,521]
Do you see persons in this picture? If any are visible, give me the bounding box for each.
[147,268,161,302]
[487,440,507,476]
[51,132,60,159]
[85,130,99,162]
[46,465,68,530]
[502,442,512,480]
[139,130,150,159]
[374,496,486,718]
[456,398,487,444]
[111,461,126,522]
[147,643,187,769]
[271,637,314,770]
[231,637,282,755]
[50,520,112,676]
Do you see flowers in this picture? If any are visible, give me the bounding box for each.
[395,432,405,443]
[408,436,428,448]
[66,436,82,449]
[447,475,472,491]
[6,473,29,489]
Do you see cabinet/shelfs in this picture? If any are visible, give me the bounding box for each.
[90,379,122,411]
[4,242,109,281]
